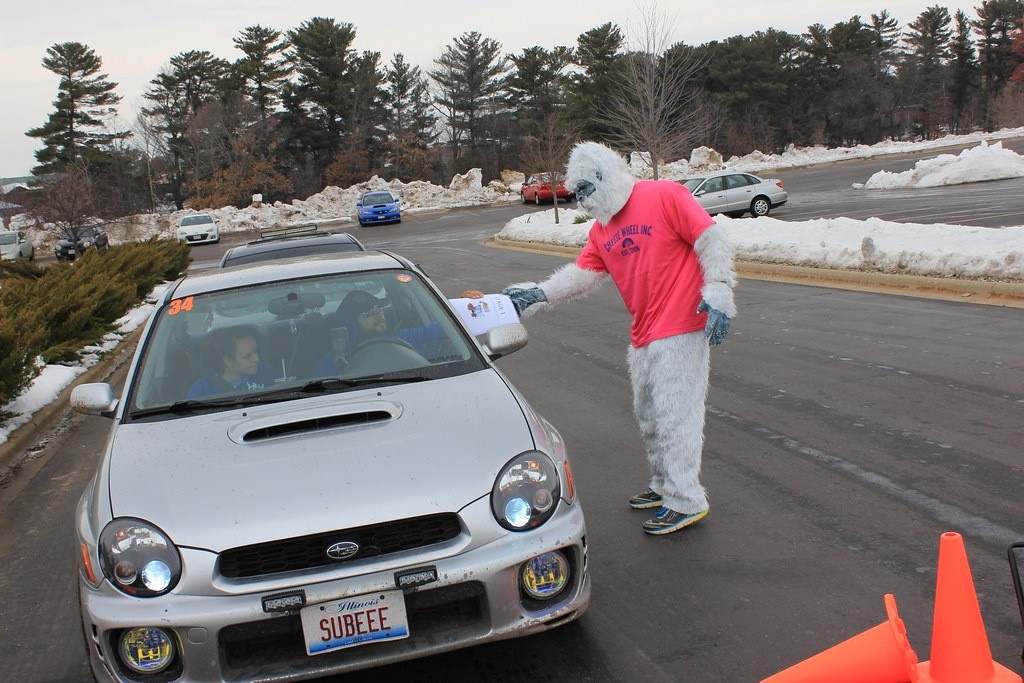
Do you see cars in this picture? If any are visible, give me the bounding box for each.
[354,190,402,226]
[0,232,34,270]
[174,214,222,247]
[521,172,576,206]
[683,171,788,218]
[53,226,109,260]
[219,226,363,268]
[67,247,595,683]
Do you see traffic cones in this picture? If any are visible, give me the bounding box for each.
[760,590,918,683]
[900,528,1024,682]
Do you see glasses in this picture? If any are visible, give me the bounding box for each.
[351,303,384,319]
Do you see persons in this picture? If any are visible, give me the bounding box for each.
[501,142,738,535]
[187,330,278,400]
[311,290,484,379]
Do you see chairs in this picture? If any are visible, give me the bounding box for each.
[175,322,287,402]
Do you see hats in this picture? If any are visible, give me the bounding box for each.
[335,290,392,318]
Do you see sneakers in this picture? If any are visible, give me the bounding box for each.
[628,490,663,509]
[642,505,709,534]
